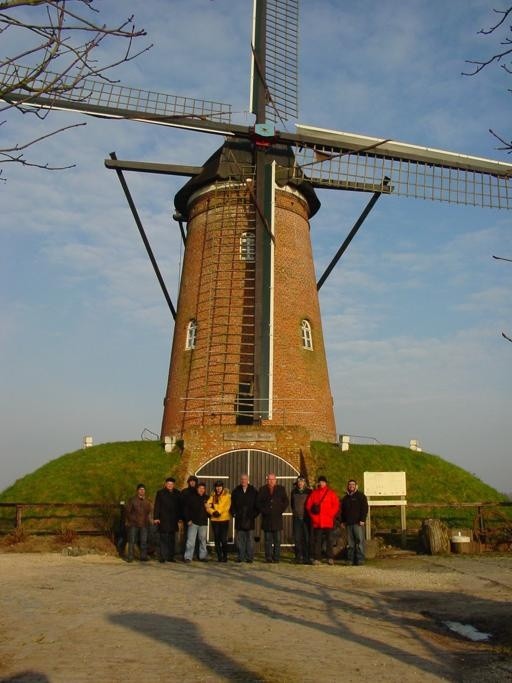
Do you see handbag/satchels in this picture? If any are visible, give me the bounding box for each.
[310,503,321,515]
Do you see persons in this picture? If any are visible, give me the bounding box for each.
[180,475,200,557]
[305,476,341,566]
[336,478,369,565]
[123,483,154,563]
[182,483,214,564]
[229,472,261,564]
[204,479,233,562]
[255,471,290,565]
[152,477,182,564]
[290,474,313,566]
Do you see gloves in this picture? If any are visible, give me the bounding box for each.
[212,511,221,518]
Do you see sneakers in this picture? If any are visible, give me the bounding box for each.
[126,555,229,563]
[236,556,365,566]
